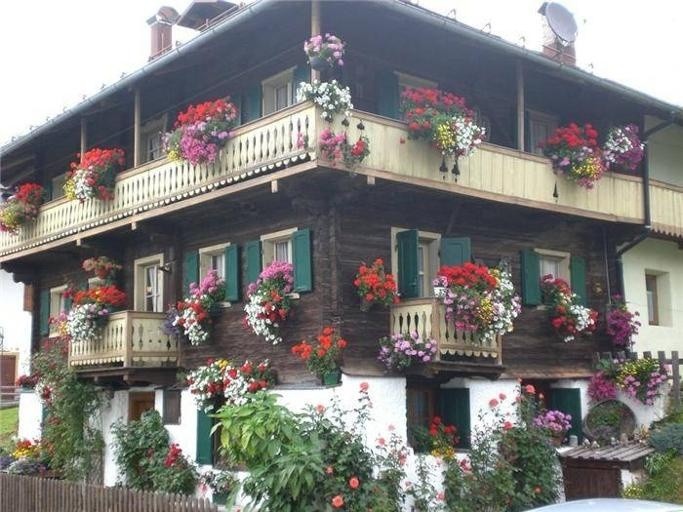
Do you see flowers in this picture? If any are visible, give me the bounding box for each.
[160,99,239,165]
[14,367,58,407]
[533,409,573,433]
[434,260,524,344]
[303,33,345,66]
[48,283,128,343]
[377,331,437,373]
[400,85,488,166]
[296,79,370,179]
[538,121,646,190]
[156,268,226,348]
[605,293,643,350]
[354,256,400,314]
[587,354,668,407]
[0,183,43,238]
[83,253,123,279]
[242,260,299,346]
[292,325,348,389]
[62,146,125,206]
[537,273,598,344]
[182,356,279,407]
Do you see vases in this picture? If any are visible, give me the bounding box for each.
[308,55,329,72]
[546,432,563,447]
